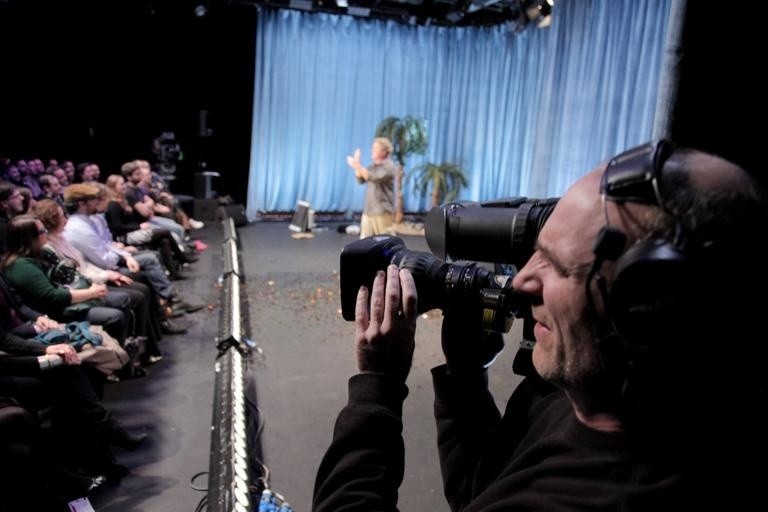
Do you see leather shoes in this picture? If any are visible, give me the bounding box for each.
[170,216,204,278]
[94,459,131,478]
[114,433,149,450]
[63,475,106,501]
[127,287,202,378]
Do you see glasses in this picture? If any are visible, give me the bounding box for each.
[38,228,48,234]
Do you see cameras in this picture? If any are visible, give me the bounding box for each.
[340,231,529,339]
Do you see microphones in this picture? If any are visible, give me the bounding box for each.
[584,225,629,312]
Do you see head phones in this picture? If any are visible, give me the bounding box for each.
[585,139,710,351]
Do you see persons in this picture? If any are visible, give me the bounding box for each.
[311,139,767,508]
[345,137,399,241]
[1,151,208,512]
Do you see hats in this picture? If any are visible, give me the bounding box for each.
[64,184,101,202]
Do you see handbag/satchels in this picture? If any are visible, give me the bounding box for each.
[55,269,106,315]
